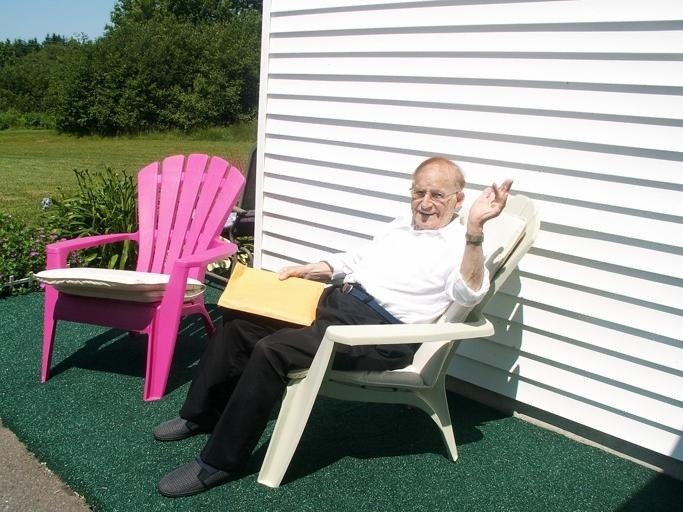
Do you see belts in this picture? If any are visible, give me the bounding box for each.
[340,283,422,350]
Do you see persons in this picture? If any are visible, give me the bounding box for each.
[152,157,514,498]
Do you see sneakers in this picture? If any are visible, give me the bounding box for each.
[154,416,211,440]
[158,454,239,497]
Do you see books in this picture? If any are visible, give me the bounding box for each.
[215,260,327,328]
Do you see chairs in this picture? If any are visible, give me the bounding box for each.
[40,154,246,402]
[255,192,543,490]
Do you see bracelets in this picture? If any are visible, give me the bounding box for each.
[465,232,484,246]
